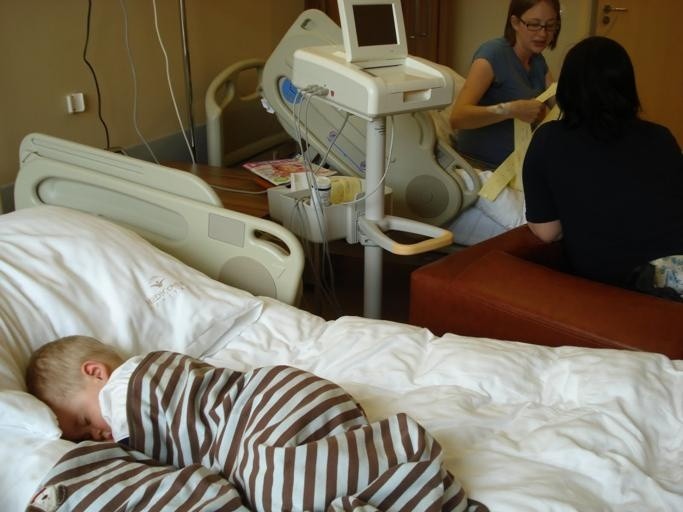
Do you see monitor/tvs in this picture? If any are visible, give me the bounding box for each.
[338,0,409,61]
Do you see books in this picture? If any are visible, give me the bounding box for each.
[243,154,338,185]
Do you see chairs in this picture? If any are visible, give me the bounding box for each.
[410,223,683,361]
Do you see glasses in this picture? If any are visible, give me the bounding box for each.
[518,16,560,32]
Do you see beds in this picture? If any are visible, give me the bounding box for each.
[1,134,681,511]
[260,9,524,255]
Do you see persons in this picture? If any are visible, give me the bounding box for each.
[450,0,562,167]
[523,37,682,301]
[26,334,149,443]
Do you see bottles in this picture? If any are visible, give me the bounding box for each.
[309,176,331,207]
